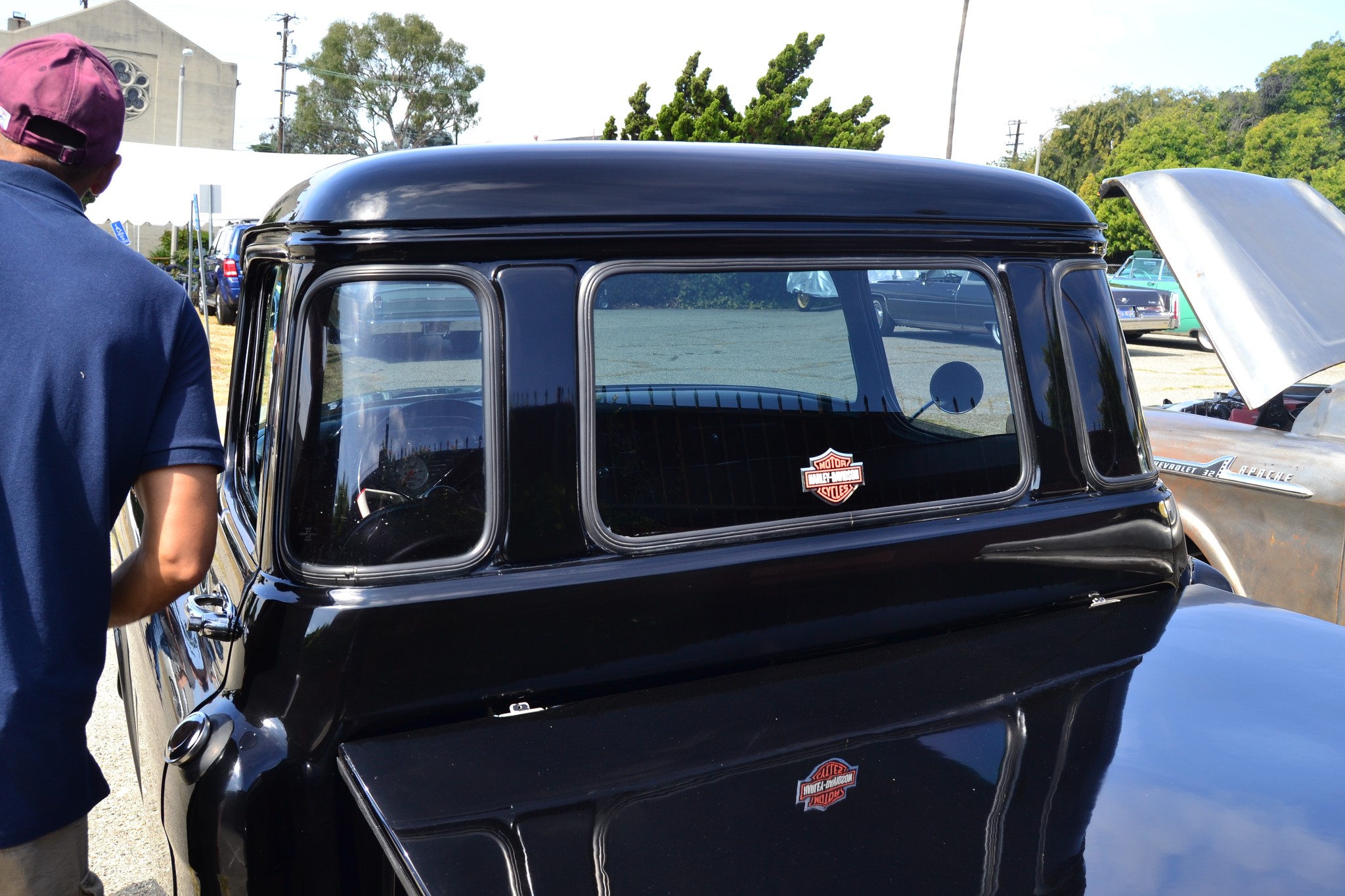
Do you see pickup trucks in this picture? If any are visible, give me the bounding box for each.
[103,136,1345,896]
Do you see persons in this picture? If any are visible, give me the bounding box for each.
[156,259,169,268]
[1,35,225,895]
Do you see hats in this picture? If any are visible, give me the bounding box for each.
[0,32,124,162]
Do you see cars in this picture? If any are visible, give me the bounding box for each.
[785,253,1215,354]
[179,217,486,354]
[1094,166,1345,508]
